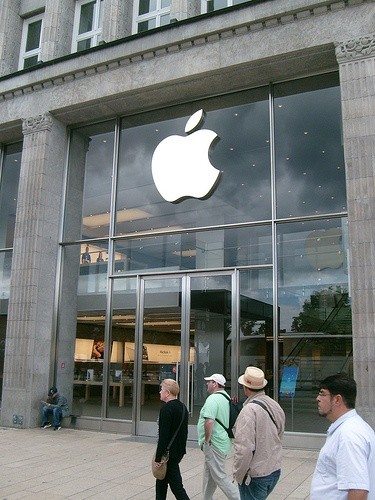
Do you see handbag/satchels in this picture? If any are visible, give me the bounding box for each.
[152,450,170,480]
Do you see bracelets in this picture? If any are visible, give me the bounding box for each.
[48,404,50,407]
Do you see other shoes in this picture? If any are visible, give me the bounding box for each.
[54,425,61,431]
[41,423,51,429]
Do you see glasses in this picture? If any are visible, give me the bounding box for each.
[316,392,332,397]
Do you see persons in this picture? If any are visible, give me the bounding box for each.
[198,373,239,500]
[232,366,285,500]
[152,378,191,500]
[310,373,374,500]
[40,388,69,430]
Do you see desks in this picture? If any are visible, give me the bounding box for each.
[73,379,159,408]
[80,256,148,272]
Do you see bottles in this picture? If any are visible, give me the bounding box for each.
[232,393,238,403]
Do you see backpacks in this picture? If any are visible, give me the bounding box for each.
[214,391,243,438]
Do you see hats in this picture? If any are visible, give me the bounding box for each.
[204,373,227,387]
[48,387,57,396]
[238,366,268,390]
